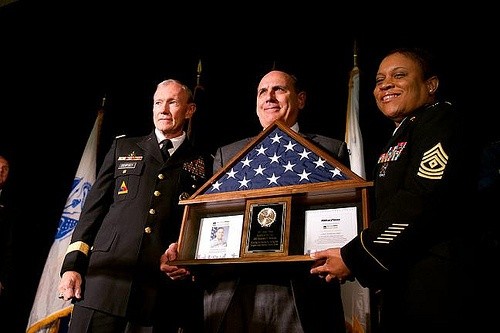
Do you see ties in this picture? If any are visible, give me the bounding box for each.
[158,139,174,162]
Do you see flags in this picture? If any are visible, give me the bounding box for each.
[25,111,102,333]
[339,66,371,332]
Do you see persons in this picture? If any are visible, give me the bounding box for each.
[59,78,208,333]
[308,51,500,333]
[0,156,30,291]
[158,70,350,333]
